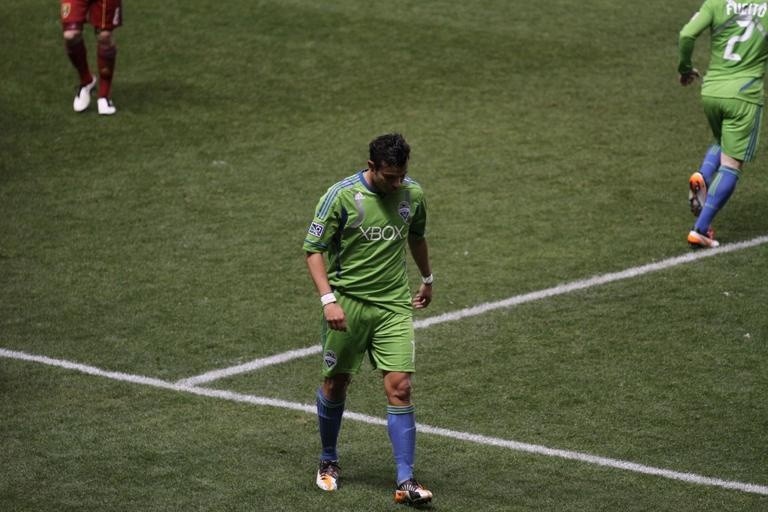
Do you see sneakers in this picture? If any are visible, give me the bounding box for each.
[316,458,341,491]
[688,172,707,217]
[73,73,96,112]
[395,478,433,505]
[96,97,117,115]
[687,228,720,249]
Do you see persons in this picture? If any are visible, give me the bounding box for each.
[299,133,433,504]
[57,0,121,118]
[677,1,768,248]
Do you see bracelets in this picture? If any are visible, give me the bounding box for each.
[421,273,433,284]
[320,292,335,307]
